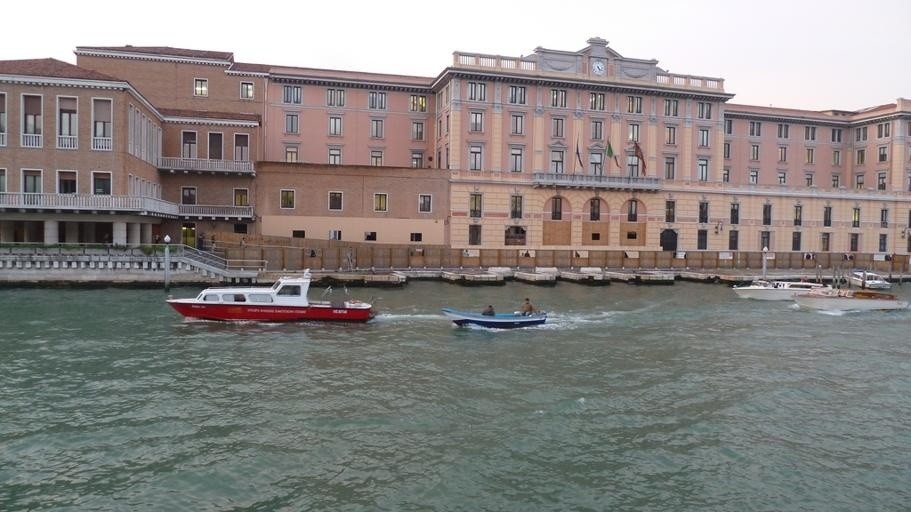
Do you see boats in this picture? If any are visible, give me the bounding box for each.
[731,271,910,310]
[441,305,549,329]
[163,267,375,323]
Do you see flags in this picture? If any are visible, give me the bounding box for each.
[575,139,583,168]
[606,141,621,168]
[635,143,646,176]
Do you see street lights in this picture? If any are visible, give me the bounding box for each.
[163,234,173,289]
[761,247,769,280]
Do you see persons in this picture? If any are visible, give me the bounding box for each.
[240,237,247,248]
[197,233,204,250]
[482,305,495,316]
[155,233,159,243]
[520,298,532,316]
[211,233,216,253]
